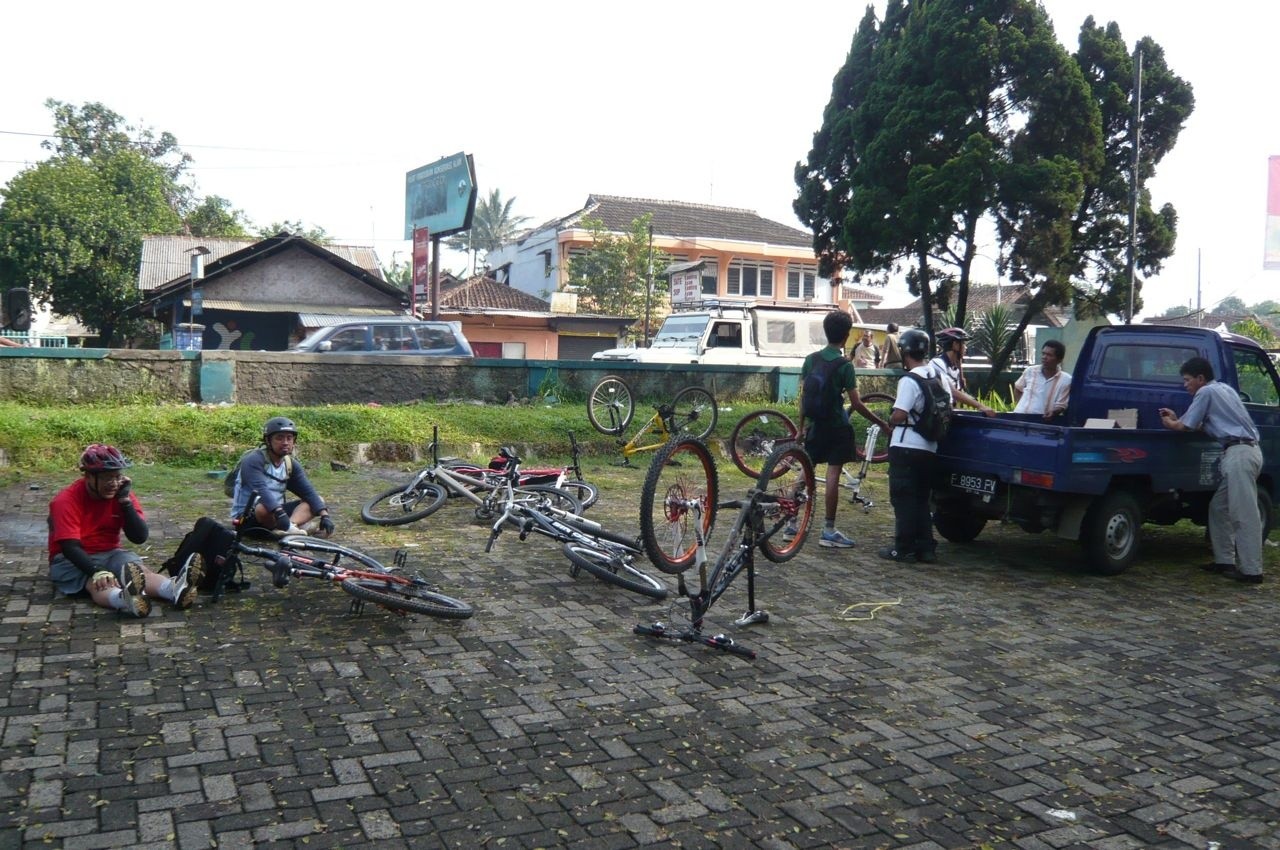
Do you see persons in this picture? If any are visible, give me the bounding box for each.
[844,329,880,369]
[336,333,353,350]
[1158,357,1264,584]
[48,444,207,617]
[0,336,25,347]
[231,416,334,536]
[1013,339,1072,420]
[882,324,903,369]
[879,327,995,563]
[783,311,892,547]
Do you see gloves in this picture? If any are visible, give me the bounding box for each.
[319,515,334,536]
[274,509,290,531]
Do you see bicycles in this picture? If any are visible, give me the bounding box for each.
[586,377,719,462]
[361,424,667,600]
[730,394,908,513]
[636,435,815,658]
[197,487,478,618]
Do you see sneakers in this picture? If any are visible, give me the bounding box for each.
[818,529,855,547]
[782,527,798,541]
[114,562,152,617]
[171,553,206,609]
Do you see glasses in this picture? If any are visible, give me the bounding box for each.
[90,473,123,484]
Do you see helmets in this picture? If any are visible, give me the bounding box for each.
[79,443,131,470]
[935,327,974,347]
[263,417,298,443]
[896,329,930,356]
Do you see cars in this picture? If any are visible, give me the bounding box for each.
[287,314,477,357]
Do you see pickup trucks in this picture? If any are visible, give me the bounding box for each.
[924,321,1280,573]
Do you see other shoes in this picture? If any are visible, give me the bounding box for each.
[919,548,936,561]
[1198,561,1237,575]
[300,521,317,536]
[1223,569,1264,583]
[879,547,918,563]
[273,522,308,536]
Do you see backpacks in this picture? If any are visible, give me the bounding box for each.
[896,371,952,440]
[225,449,292,498]
[801,351,849,419]
[163,516,238,595]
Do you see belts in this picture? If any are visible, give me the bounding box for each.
[1223,438,1259,450]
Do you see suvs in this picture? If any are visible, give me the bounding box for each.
[591,301,840,367]
